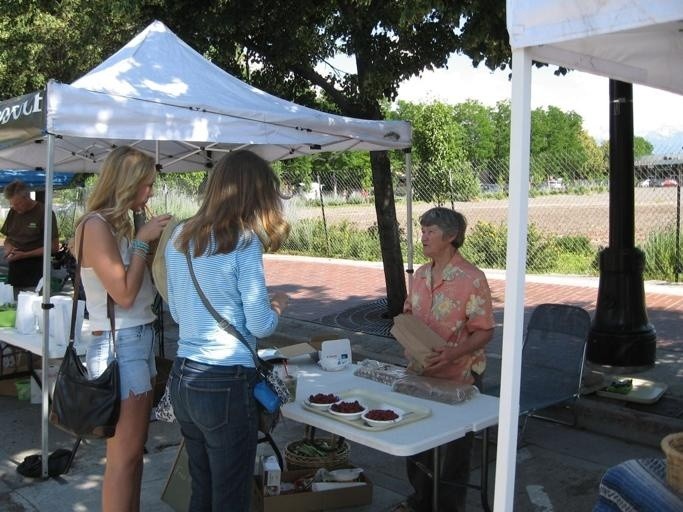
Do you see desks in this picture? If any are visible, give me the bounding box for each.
[260,354,500,512]
[593,458,683,512]
[0,318,149,474]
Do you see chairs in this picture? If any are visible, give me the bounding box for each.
[472,303,608,450]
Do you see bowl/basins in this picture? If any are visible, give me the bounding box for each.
[0,310,16,327]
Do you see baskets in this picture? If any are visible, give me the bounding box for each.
[284,435,352,471]
[660,431,682,494]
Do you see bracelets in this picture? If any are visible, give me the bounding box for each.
[130,239,150,263]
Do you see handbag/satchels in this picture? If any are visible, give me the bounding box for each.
[253,363,291,436]
[47,345,122,440]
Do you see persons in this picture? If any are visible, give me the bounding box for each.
[72,145,181,510]
[161,148,290,512]
[0,180,59,305]
[388,207,496,512]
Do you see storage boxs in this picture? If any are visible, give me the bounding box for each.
[250,465,373,512]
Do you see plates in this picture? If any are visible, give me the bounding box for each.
[361,409,401,427]
[304,395,345,410]
[328,467,361,482]
[328,404,369,421]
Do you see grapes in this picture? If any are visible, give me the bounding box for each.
[309,392,339,404]
[330,401,362,413]
[366,409,398,420]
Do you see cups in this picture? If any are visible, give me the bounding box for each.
[16,379,31,400]
[276,365,298,402]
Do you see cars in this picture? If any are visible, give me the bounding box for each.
[473,177,681,197]
